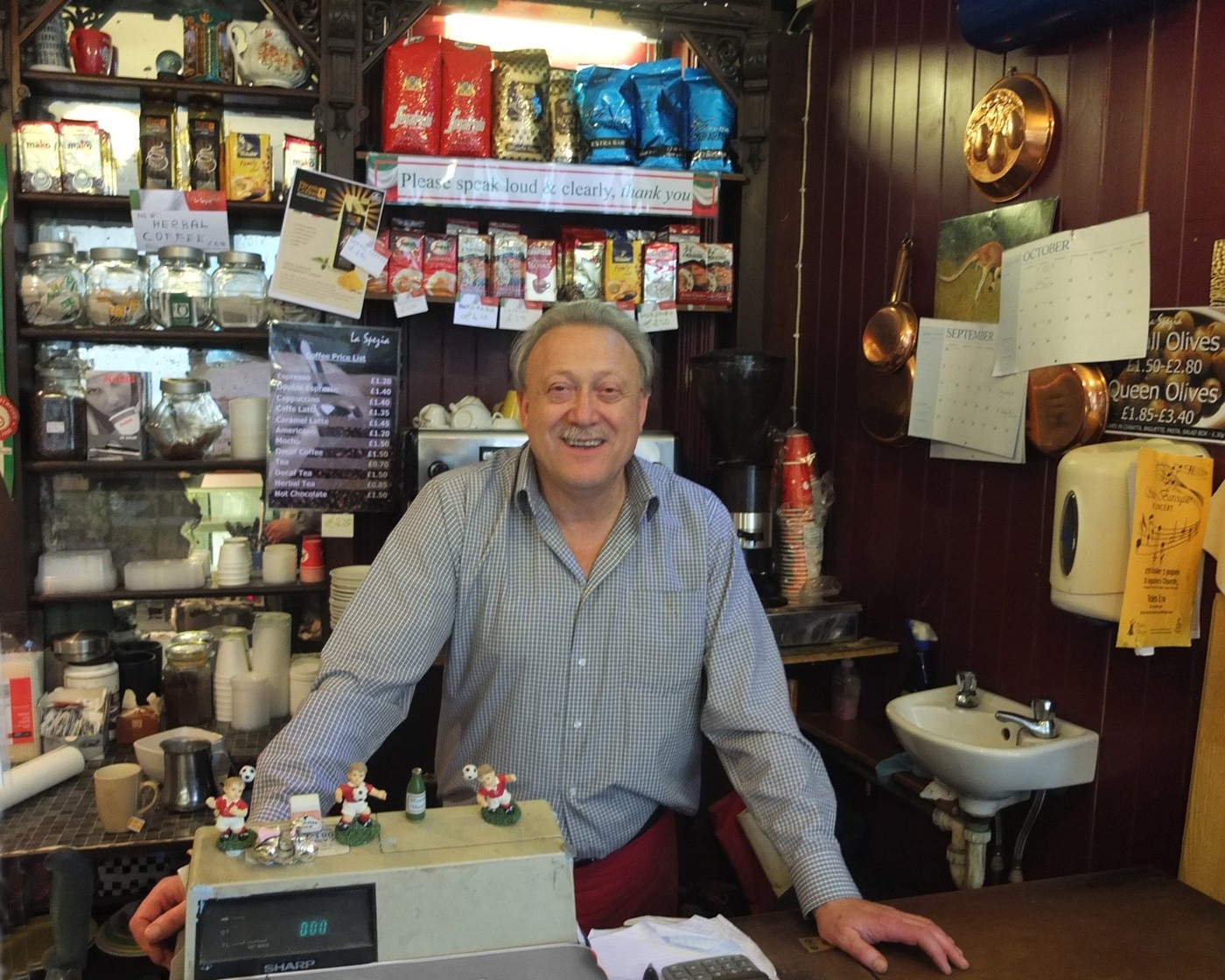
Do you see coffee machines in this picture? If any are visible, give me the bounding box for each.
[689,349,788,608]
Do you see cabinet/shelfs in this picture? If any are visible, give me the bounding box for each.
[0,0,751,980]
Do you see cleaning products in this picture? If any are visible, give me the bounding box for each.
[898,619,940,691]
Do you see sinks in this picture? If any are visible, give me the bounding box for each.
[886,684,1100,800]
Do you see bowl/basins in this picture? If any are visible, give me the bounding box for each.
[963,74,1054,204]
[34,551,118,596]
[130,726,225,788]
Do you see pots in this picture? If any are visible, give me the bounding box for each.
[855,235,922,450]
[1027,363,1109,458]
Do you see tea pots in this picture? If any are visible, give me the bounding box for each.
[226,13,314,89]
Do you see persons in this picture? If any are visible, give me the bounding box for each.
[334,762,387,831]
[131,300,970,975]
[476,764,517,815]
[205,777,250,841]
[264,507,333,545]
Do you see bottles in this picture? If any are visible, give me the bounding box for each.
[144,379,227,459]
[164,642,213,727]
[30,363,86,463]
[17,240,323,329]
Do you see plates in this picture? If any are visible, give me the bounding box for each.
[330,564,369,632]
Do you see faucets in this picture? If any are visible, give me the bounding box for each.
[955,670,979,709]
[993,698,1058,739]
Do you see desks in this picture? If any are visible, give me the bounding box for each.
[749,863,1224,980]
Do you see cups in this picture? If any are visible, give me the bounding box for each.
[94,762,158,832]
[69,30,113,77]
[775,434,826,605]
[115,652,156,707]
[197,531,325,729]
[411,390,521,431]
[157,741,217,812]
